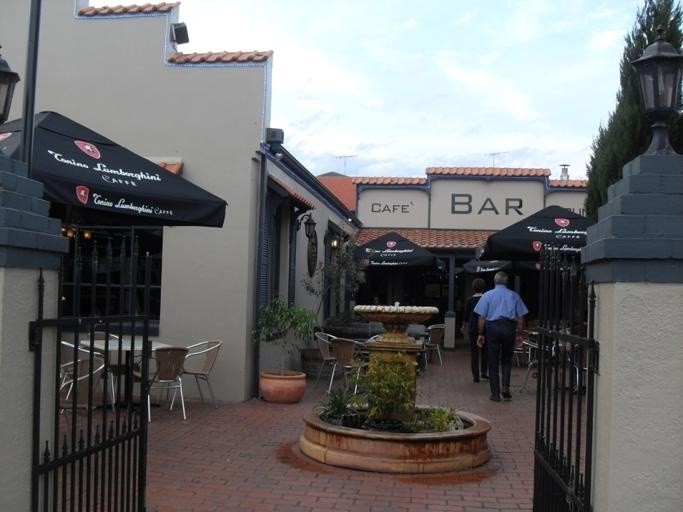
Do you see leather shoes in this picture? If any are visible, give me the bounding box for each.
[489,393,501,401]
[501,391,512,400]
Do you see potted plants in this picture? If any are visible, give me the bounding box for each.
[251,289,318,404]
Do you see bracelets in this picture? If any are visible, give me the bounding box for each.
[479,332,484,335]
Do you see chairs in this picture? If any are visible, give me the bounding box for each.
[59,329,224,423]
[314,331,371,403]
[513,326,594,394]
[423,323,448,369]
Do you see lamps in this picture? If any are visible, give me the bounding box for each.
[296,211,317,240]
[323,228,341,252]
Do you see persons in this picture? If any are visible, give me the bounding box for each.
[472,270,530,402]
[463,278,490,383]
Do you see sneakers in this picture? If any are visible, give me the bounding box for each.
[481,376,490,380]
